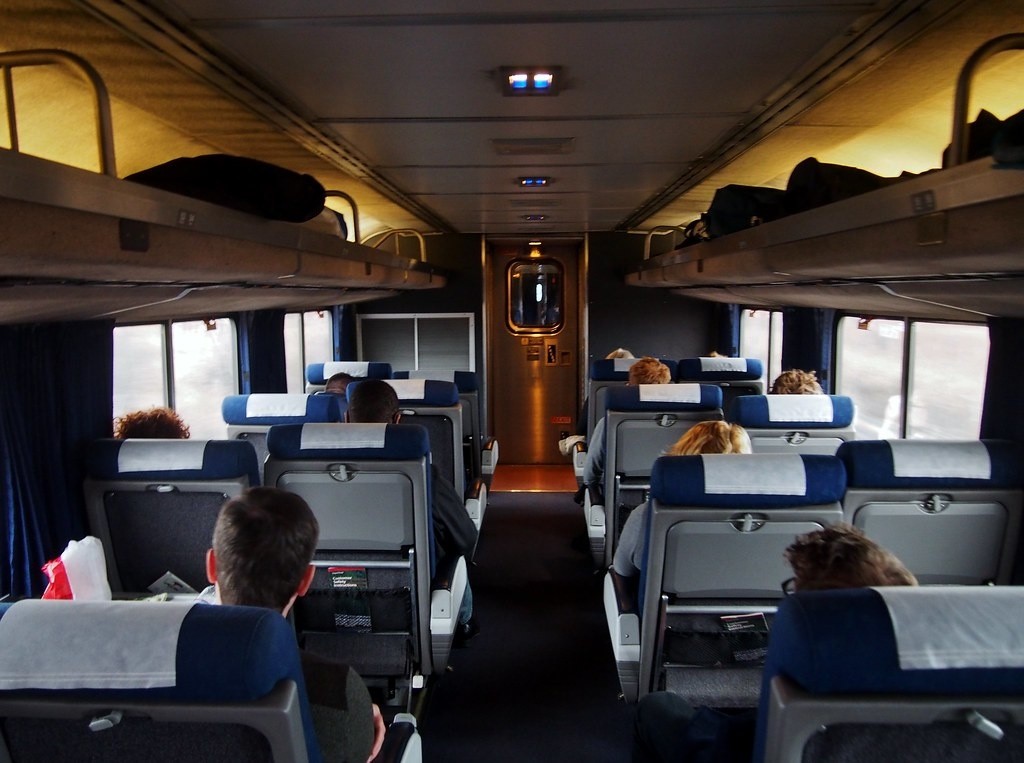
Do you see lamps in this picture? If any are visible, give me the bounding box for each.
[516,175,554,187]
[497,65,563,98]
[522,214,548,220]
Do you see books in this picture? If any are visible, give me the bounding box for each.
[327,567,372,633]
[147,571,198,593]
[720,612,770,664]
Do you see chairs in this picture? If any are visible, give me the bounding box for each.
[573,358,677,491]
[753,585,1024,763]
[724,394,855,454]
[603,452,847,707]
[264,421,468,707]
[346,379,487,531]
[223,394,345,486]
[305,361,391,395]
[77,437,260,602]
[835,439,1024,586]
[0,599,325,763]
[390,370,498,497]
[583,383,724,573]
[676,357,765,423]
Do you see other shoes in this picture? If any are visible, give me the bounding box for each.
[574,484,588,507]
[451,618,476,650]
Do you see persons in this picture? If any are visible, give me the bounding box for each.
[573,348,636,436]
[114,408,191,439]
[767,369,824,394]
[781,527,919,591]
[206,486,386,763]
[613,421,753,577]
[344,379,478,552]
[582,356,671,486]
[314,373,354,422]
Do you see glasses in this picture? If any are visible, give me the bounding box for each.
[781,577,798,596]
[768,387,773,392]
